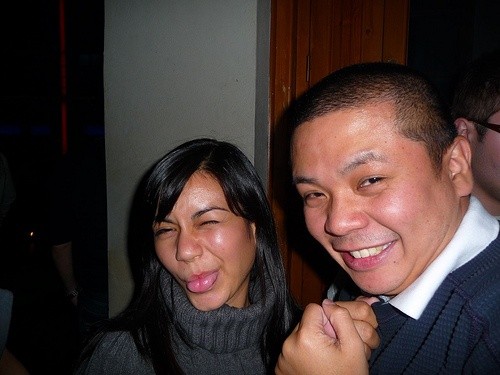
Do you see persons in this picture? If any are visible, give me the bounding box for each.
[0,138,304,375]
[268,49,500,375]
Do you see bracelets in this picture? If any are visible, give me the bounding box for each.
[70,289,80,299]
